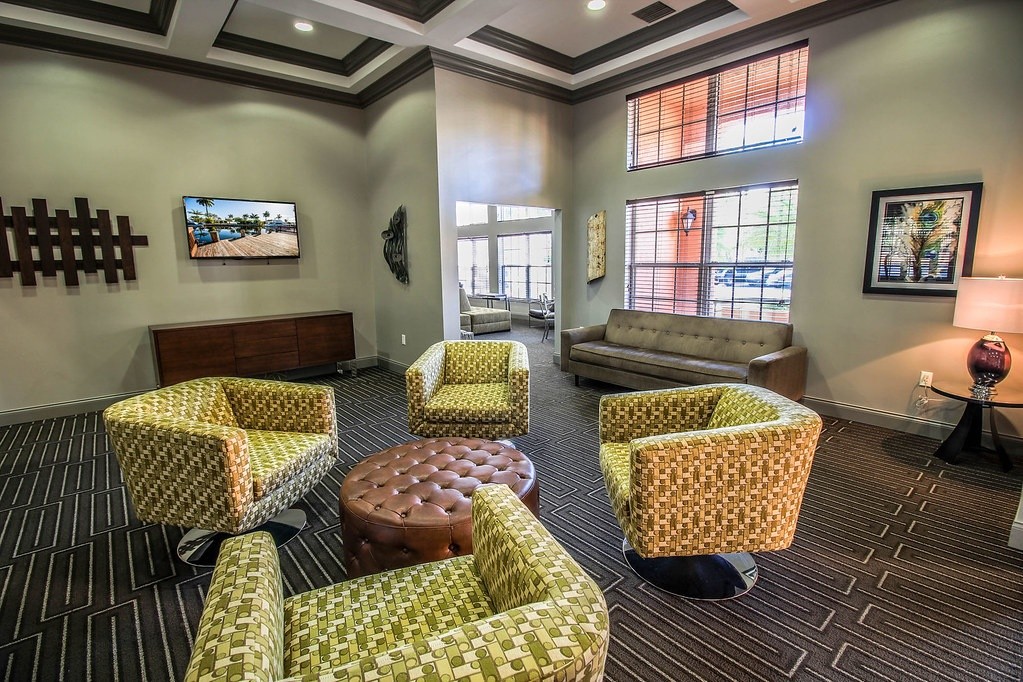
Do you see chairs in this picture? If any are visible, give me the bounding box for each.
[528,300,550,329]
[539,295,555,344]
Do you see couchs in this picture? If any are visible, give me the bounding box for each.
[104,375,339,569]
[185,481,612,682]
[458,282,511,334]
[559,308,808,413]
[597,382,824,602]
[403,337,531,437]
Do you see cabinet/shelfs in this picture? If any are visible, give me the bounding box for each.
[149,309,359,387]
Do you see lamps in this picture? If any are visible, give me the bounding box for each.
[952,275,1023,400]
[294,19,314,32]
[586,0,607,11]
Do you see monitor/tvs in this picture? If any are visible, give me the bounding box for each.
[182,195,300,260]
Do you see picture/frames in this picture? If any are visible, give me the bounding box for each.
[861,183,983,294]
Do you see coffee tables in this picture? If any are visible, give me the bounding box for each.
[931,381,1023,468]
[337,438,540,577]
